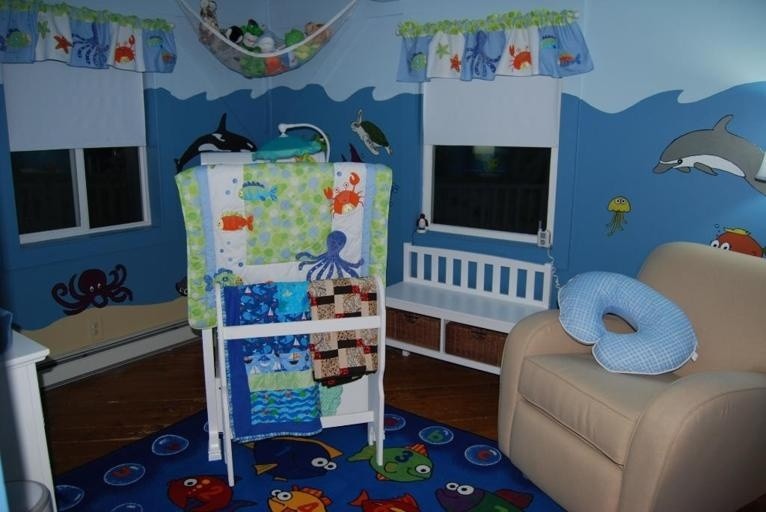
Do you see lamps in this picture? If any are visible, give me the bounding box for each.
[252,123,330,163]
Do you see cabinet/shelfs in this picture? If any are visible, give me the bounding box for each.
[173,163,393,489]
[0,332,60,512]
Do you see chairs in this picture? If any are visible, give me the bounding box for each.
[498,241,765,512]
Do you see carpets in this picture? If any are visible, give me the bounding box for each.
[56,394,558,512]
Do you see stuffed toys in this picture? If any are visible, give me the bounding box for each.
[198,0,331,78]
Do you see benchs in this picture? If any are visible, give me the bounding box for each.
[385,242,553,376]
